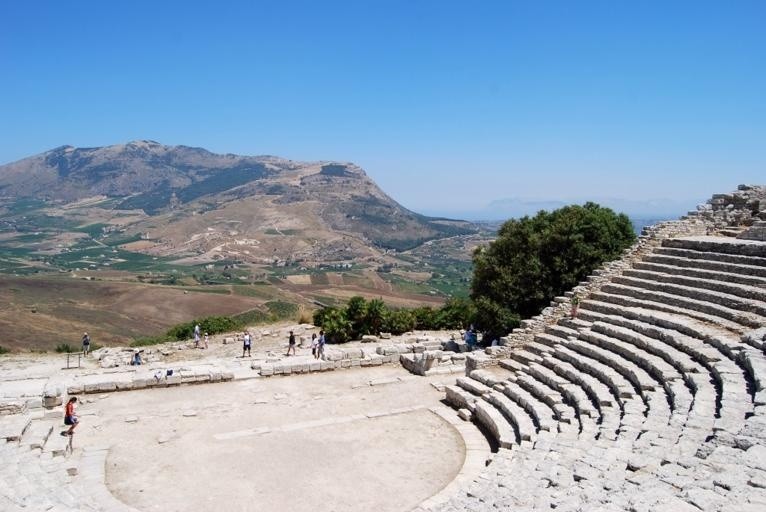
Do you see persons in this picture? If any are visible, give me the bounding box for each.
[131,348,143,373]
[310,334,318,359]
[444,325,498,353]
[568,295,581,320]
[316,330,325,360]
[239,331,252,357]
[64,396,80,436]
[82,331,90,357]
[286,331,296,356]
[203,333,209,349]
[193,323,201,346]
